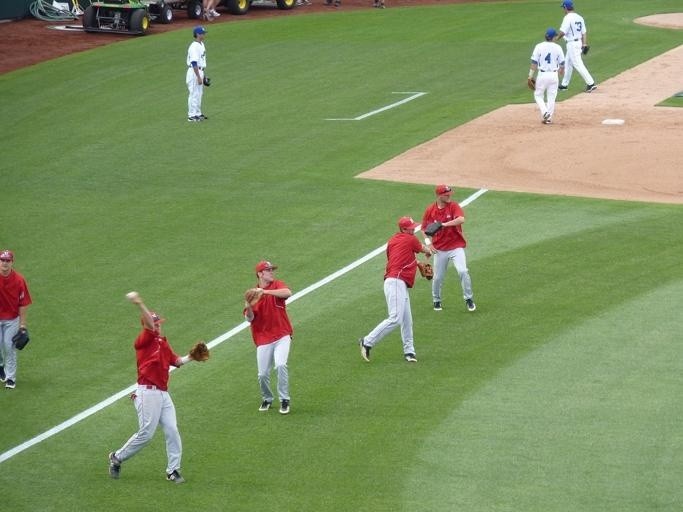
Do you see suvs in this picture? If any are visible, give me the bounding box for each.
[62,1,293,36]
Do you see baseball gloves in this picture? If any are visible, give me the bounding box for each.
[581,46,590,54]
[245,287,264,307]
[12,328,29,350]
[527,77,535,89]
[203,77,210,86]
[425,221,441,236]
[190,341,208,363]
[418,262,433,280]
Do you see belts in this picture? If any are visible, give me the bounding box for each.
[147,385,159,389]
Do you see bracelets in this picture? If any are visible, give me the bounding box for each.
[255,286,264,297]
[425,238,432,246]
[18,322,28,331]
[180,355,192,366]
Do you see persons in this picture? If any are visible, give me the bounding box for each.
[108,289,208,485]
[202,0,223,21]
[242,258,294,416]
[359,214,433,362]
[321,0,342,7]
[421,183,477,313]
[526,28,567,123]
[371,0,388,10]
[556,0,597,94]
[184,25,213,123]
[0,249,30,390]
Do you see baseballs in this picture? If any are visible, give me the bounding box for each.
[126,291,138,301]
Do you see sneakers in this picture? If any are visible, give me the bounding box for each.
[259,400,291,414]
[432,301,443,311]
[357,338,374,364]
[0,366,7,382]
[109,452,120,478]
[188,114,206,121]
[5,380,15,389]
[404,353,418,363]
[464,298,476,311]
[167,470,185,483]
[542,84,597,124]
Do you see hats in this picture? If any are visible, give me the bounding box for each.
[0,250,13,260]
[545,28,558,37]
[193,26,208,34]
[561,0,573,7]
[435,185,454,196]
[141,311,165,325]
[399,216,420,229]
[256,261,278,272]
